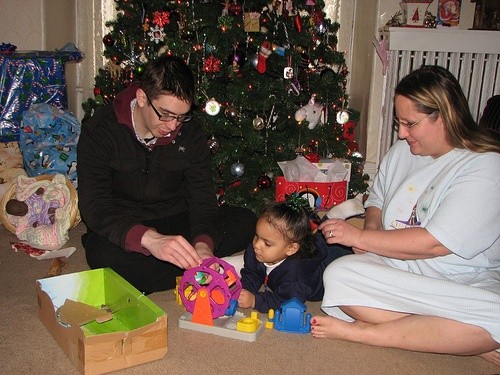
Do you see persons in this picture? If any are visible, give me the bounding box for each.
[311,66,500,366]
[238,195,355,314]
[77,55,257,296]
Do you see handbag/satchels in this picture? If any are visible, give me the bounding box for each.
[276,174,349,231]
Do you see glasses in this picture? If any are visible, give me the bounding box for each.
[392,110,436,129]
[145,93,194,122]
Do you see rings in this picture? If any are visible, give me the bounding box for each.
[329,231,333,237]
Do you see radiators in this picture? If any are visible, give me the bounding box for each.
[377,26,500,173]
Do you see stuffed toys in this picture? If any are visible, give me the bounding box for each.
[295,97,323,130]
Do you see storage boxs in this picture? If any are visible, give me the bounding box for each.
[36,267,168,375]
[275,156,352,212]
